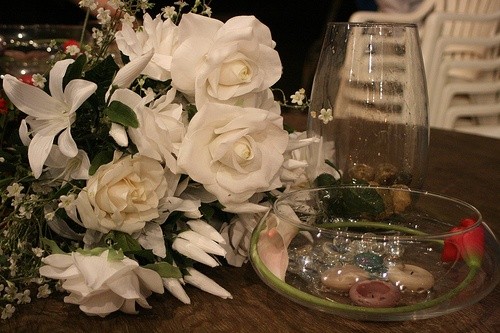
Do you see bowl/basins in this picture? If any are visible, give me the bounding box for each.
[249,183,500,322]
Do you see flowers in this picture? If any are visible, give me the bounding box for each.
[0,0,384,319]
[252,216,484,312]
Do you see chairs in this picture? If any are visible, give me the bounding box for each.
[333,0,500,139]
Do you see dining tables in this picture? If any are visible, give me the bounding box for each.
[0,113,499,333]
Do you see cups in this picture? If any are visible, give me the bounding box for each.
[306,20,430,225]
